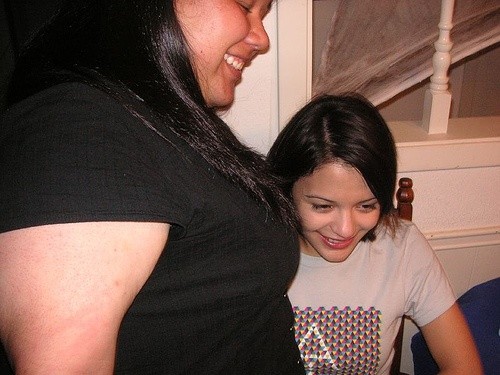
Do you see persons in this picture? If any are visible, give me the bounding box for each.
[0,0,308,375]
[263,90,485,375]
